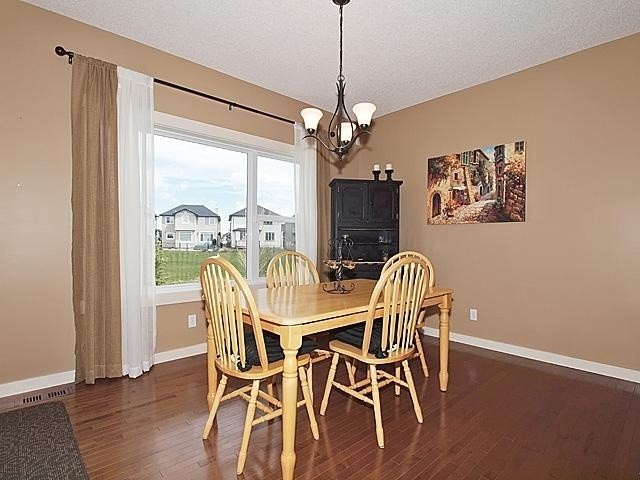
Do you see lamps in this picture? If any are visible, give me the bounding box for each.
[300,0,376,162]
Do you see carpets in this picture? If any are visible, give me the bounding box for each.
[0,402,89,480]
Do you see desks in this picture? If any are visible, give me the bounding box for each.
[202,278,453,480]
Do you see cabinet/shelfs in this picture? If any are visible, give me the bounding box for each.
[329,179,403,281]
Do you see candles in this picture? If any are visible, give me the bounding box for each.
[385,164,392,171]
[374,165,379,171]
